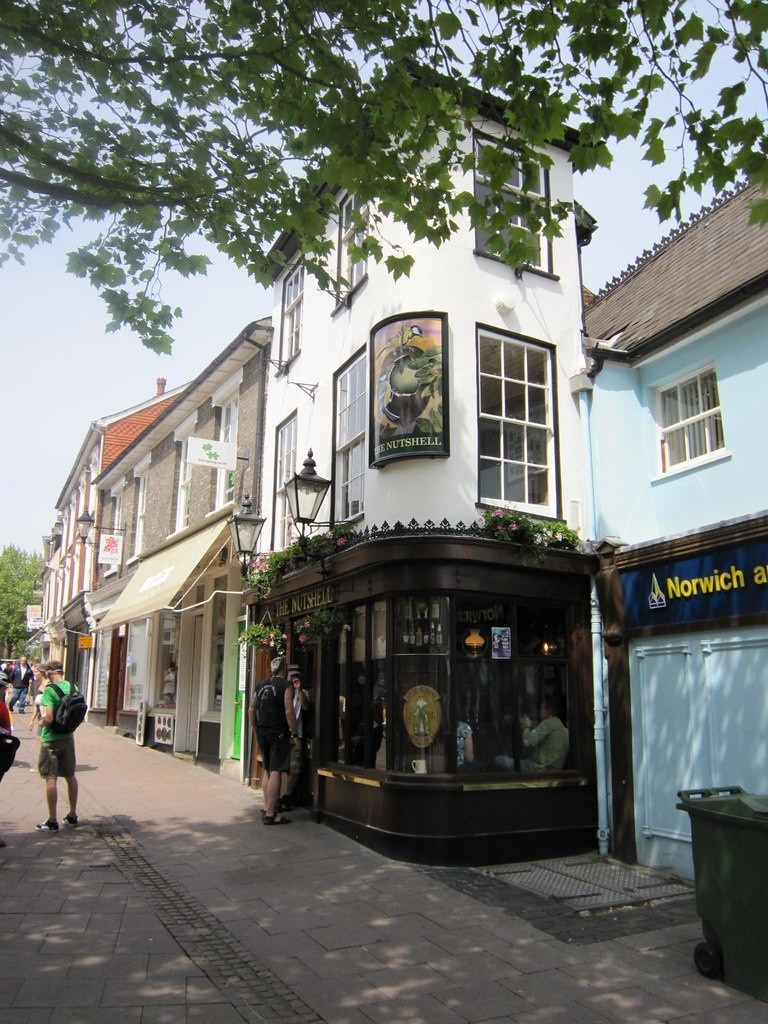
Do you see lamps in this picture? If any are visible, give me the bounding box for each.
[77,510,127,545]
[284,449,333,581]
[225,493,267,582]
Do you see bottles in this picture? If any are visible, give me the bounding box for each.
[402,619,443,645]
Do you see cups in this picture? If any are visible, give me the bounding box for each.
[411,759,427,774]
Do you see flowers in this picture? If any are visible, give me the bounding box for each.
[236,621,288,656]
[293,608,343,646]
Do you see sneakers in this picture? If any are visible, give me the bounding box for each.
[63,814,78,827]
[35,819,59,832]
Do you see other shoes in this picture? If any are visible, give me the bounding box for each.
[282,799,294,810]
[19,710,25,714]
[9,704,14,712]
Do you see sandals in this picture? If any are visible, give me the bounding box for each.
[262,810,290,825]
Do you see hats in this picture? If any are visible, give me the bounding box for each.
[288,664,303,677]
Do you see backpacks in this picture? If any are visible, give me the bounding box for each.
[47,681,88,735]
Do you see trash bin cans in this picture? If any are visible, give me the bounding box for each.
[676,783,768,1003]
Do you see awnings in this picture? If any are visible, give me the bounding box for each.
[27,600,90,647]
[89,516,246,632]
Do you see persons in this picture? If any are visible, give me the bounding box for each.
[457,699,487,772]
[215,677,222,699]
[338,695,346,751]
[30,664,51,773]
[165,663,177,699]
[248,657,311,825]
[0,671,11,847]
[0,656,41,714]
[35,661,79,832]
[160,693,175,709]
[495,701,569,770]
